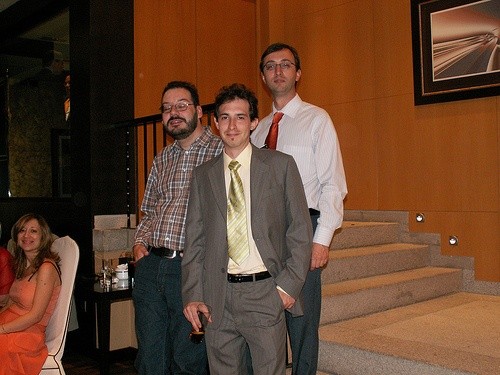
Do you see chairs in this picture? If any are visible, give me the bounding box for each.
[38,236,80,375]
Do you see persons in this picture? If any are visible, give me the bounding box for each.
[0,213,62,375]
[132,81,224,375]
[181,83,313,375]
[250,43,348,375]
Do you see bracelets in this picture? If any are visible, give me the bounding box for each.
[2,324,7,333]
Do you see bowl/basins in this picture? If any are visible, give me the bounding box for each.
[116,270,128,279]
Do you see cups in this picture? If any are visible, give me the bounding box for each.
[103,259,112,287]
[190,304,213,344]
[128,260,135,286]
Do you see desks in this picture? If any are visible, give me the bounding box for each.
[94,283,137,375]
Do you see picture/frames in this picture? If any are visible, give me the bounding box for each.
[409,0,500,106]
[51,127,74,199]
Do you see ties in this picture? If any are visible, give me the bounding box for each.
[265,112,283,149]
[227,160,251,266]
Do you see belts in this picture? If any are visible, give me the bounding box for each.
[227,271,271,284]
[152,246,181,259]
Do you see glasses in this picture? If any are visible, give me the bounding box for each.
[162,101,195,113]
[264,61,296,70]
